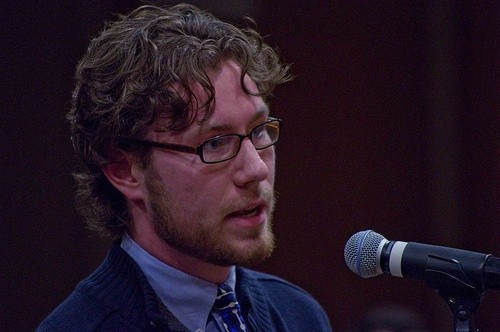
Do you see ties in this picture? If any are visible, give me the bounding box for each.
[215,282,249,332]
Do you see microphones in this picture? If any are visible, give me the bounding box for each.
[345,230,500,294]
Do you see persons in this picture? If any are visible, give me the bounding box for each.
[34,3,337,332]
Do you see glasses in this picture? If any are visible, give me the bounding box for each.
[128,115,284,164]
